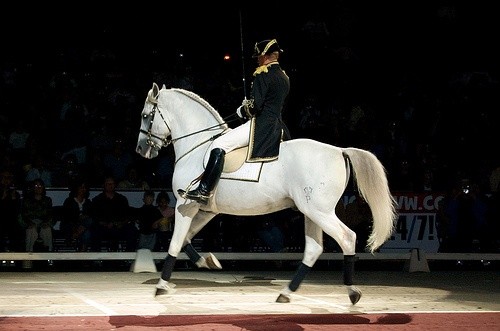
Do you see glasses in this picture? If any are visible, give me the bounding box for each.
[35,184,42,187]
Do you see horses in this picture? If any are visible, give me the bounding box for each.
[136,82,398,305]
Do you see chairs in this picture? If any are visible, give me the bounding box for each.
[36,206,203,270]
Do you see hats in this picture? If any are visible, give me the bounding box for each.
[251,38,284,57]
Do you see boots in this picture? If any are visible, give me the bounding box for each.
[177,148,227,204]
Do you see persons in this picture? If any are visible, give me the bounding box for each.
[61,180,94,252]
[177,32,290,204]
[19,182,57,251]
[90,178,130,252]
[153,192,179,252]
[133,191,164,252]
[0,171,21,264]
